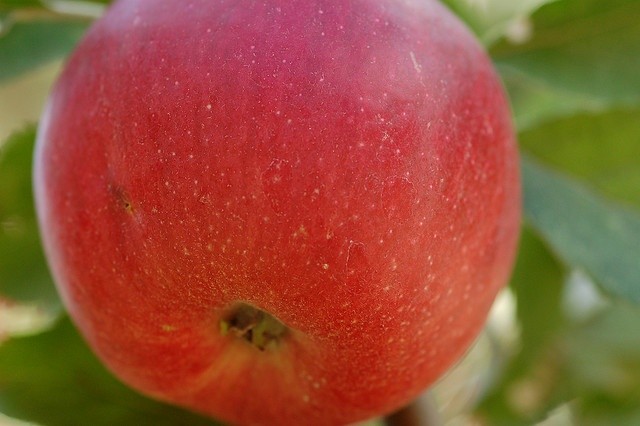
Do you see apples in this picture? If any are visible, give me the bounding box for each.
[32,1,523,426]
